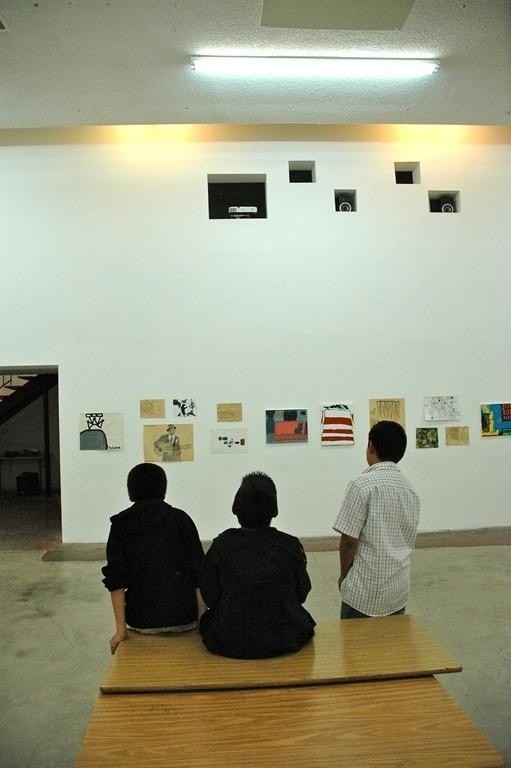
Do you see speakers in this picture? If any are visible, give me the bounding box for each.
[429,198,456,212]
[395,171,413,184]
[208,182,266,219]
[335,193,354,212]
[289,169,312,182]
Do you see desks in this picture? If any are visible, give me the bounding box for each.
[0,453,45,497]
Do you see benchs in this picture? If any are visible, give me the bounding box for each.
[99,613,464,690]
[76,675,502,768]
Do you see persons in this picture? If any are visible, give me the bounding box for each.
[197,470,320,661]
[100,462,209,656]
[332,420,423,620]
[154,423,183,462]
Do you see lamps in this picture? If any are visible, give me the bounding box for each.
[337,194,353,212]
[438,196,454,213]
